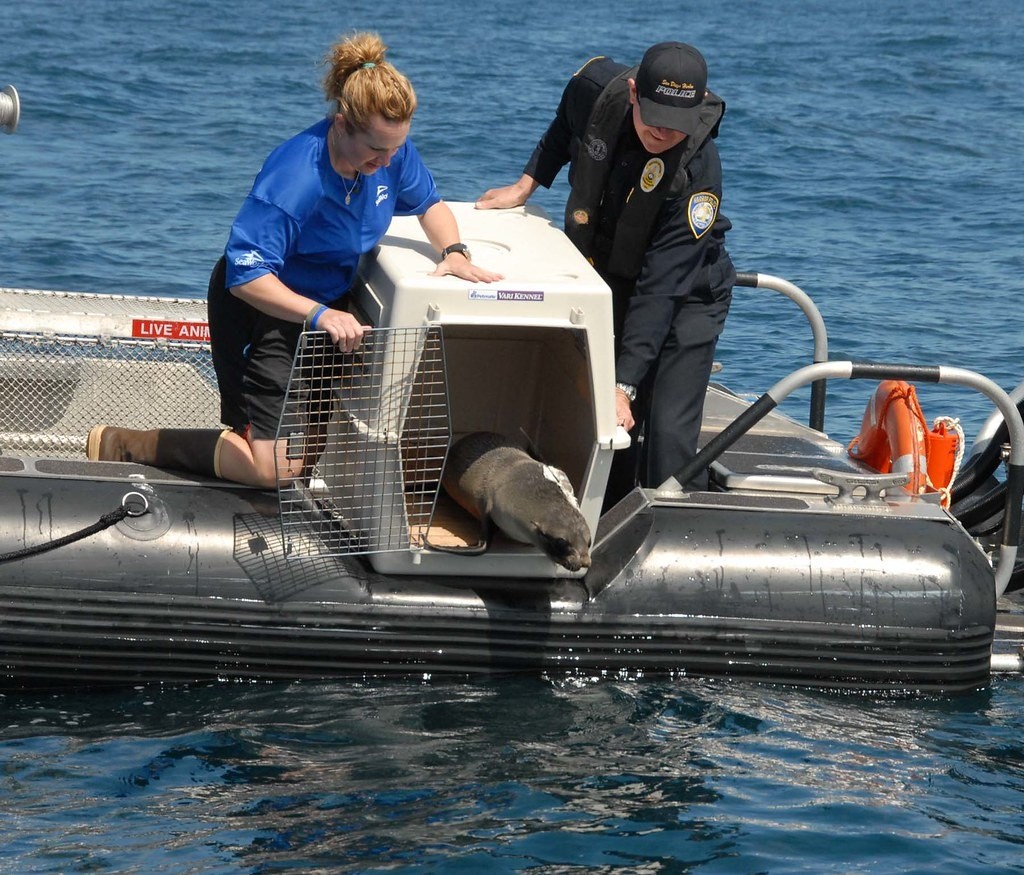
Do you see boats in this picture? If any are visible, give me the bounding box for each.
[0,268,1023,707]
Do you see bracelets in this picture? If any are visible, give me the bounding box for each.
[305,303,324,326]
[310,306,328,331]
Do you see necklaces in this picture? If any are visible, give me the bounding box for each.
[330,124,360,205]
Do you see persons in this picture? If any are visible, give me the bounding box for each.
[86,32,504,490]
[475,42,735,498]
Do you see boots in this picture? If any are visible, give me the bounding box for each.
[86,424,230,479]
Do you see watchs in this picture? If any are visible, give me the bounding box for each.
[616,382,637,402]
[441,243,471,261]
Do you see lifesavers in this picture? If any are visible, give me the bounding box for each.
[848,378,929,496]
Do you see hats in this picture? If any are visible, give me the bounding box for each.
[637,41,707,135]
